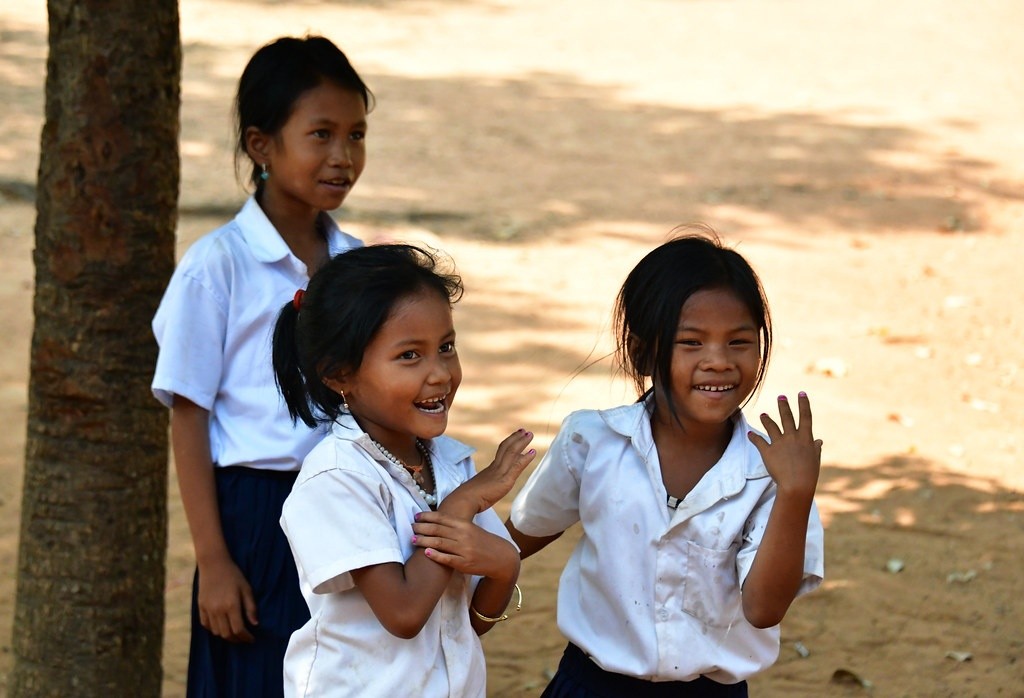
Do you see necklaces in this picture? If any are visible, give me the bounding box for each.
[668,496,682,508]
[373,440,438,506]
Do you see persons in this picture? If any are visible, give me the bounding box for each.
[149,34,372,697]
[272,245,537,697]
[502,234,825,698]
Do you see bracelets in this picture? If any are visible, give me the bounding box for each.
[471,583,522,622]
